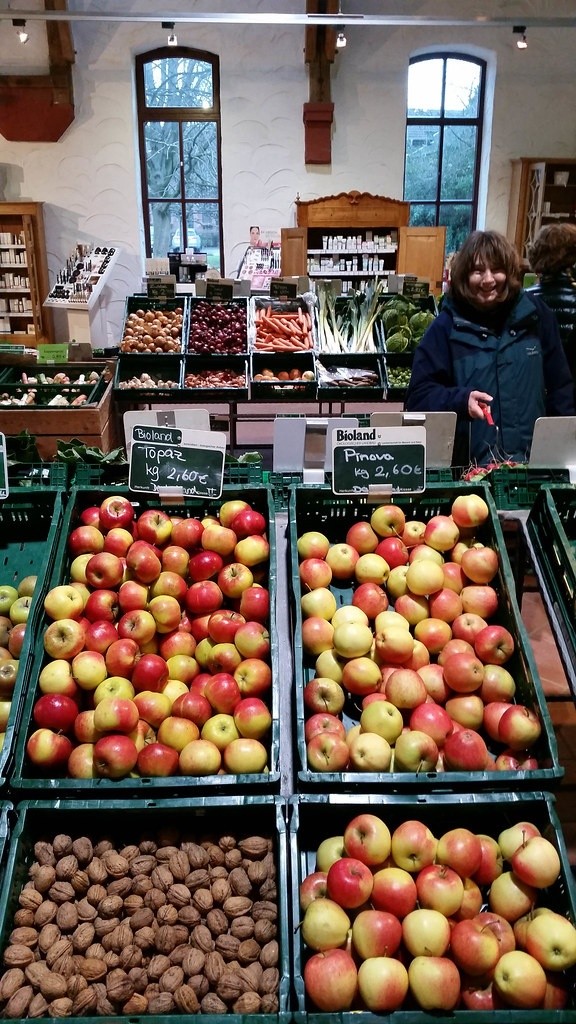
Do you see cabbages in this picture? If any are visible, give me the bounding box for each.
[382,298,437,354]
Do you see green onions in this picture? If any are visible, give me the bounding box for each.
[314,275,394,354]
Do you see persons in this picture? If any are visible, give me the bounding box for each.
[250,227,260,248]
[404,224,576,466]
[523,223,576,381]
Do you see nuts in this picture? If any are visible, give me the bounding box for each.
[0,832,281,1019]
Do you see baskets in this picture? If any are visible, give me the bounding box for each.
[0,462,576,788]
[0,364,108,409]
[0,792,576,1024]
[114,295,439,403]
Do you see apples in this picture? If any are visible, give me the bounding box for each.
[293,813,576,1011]
[294,493,543,774]
[0,574,38,759]
[27,494,274,780]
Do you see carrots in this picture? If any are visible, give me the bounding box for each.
[252,304,314,352]
